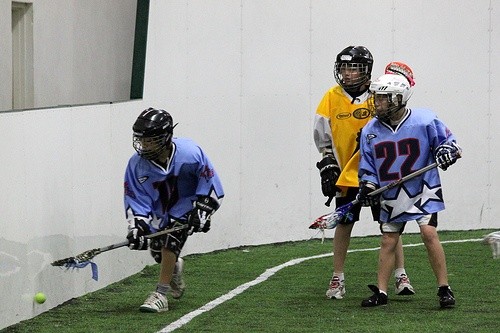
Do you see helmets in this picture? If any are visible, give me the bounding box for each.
[369,74,412,104]
[336,46,373,75]
[132,107,173,135]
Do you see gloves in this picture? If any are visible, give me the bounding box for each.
[126,217,153,251]
[435,146,456,171]
[316,158,342,198]
[186,194,220,237]
[356,182,378,207]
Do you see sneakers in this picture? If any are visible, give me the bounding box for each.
[437,286,456,306]
[395,273,415,295]
[170,258,186,299]
[326,276,346,300]
[139,292,170,313]
[361,284,388,307]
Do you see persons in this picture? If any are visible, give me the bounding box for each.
[313,45,414,299]
[123,108,225,312]
[356,62,462,309]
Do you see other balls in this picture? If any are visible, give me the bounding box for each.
[35,292,46,304]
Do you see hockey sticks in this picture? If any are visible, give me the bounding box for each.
[307,150,461,245]
[325,60,416,208]
[51,224,189,273]
[481,229,500,261]
